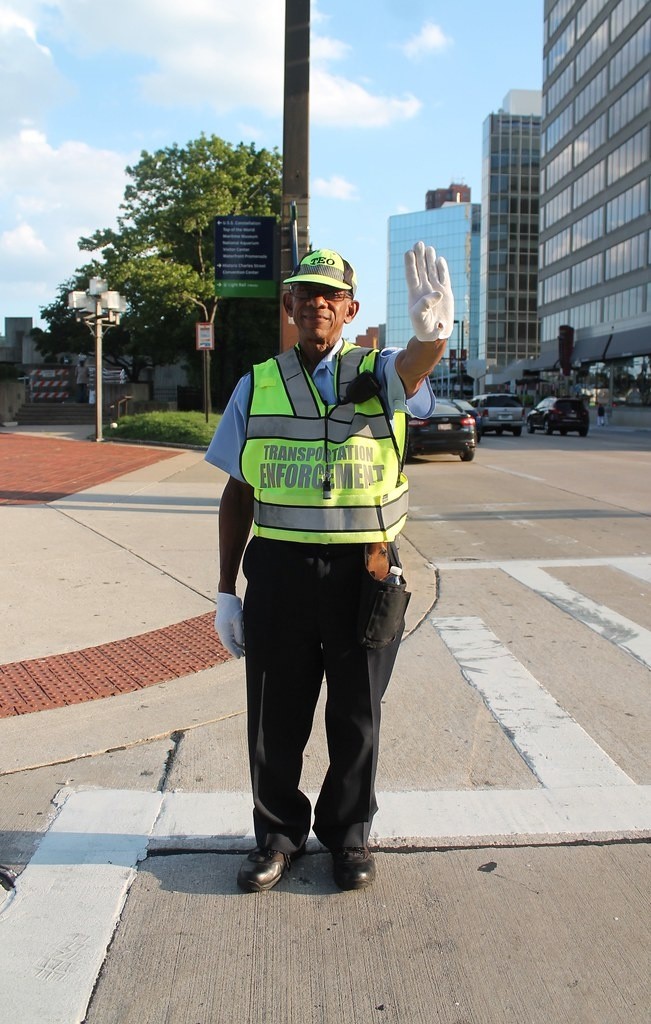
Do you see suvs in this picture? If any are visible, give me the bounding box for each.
[526,396,591,437]
[471,394,525,436]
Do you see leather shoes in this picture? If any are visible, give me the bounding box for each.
[237,845,308,892]
[332,846,378,890]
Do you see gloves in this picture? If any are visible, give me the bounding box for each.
[404,241,454,342]
[212,593,246,661]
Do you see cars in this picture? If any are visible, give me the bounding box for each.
[451,399,482,442]
[407,402,477,463]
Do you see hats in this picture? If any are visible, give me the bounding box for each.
[282,248,358,296]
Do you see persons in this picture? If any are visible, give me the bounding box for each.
[597,404,604,426]
[202,242,455,891]
[75,361,89,403]
[120,369,126,384]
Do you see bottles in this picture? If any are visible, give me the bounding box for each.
[382,565,403,586]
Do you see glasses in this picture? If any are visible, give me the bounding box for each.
[288,282,354,302]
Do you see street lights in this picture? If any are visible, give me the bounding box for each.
[455,320,460,397]
[68,277,126,441]
